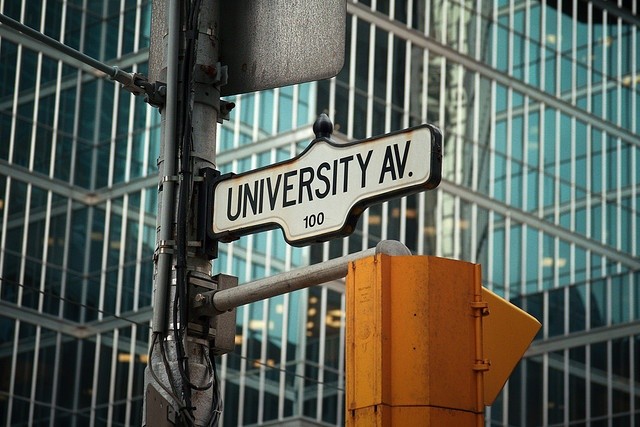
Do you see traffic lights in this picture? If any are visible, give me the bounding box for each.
[343,255,544,427]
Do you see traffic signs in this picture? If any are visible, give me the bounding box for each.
[209,112,443,247]
